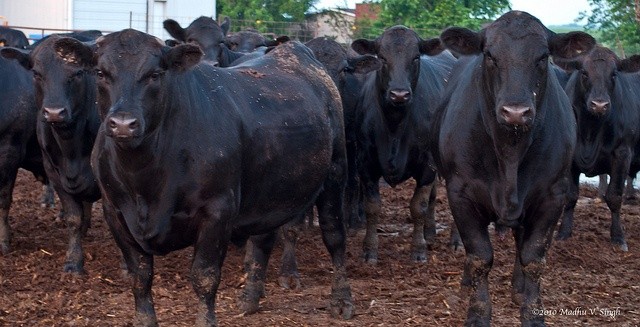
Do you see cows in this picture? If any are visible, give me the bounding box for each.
[553,44,640,253]
[263,34,384,237]
[431,10,596,327]
[222,27,290,53]
[0,34,130,284]
[0,26,30,49]
[352,24,458,265]
[0,29,103,257]
[53,28,354,327]
[161,15,271,68]
